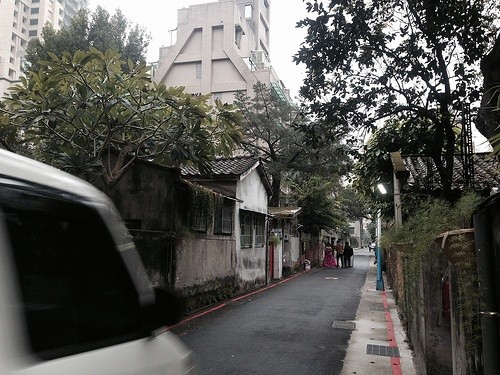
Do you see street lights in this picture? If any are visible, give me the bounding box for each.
[375,182,388,291]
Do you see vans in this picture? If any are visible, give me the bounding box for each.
[0,148,198,375]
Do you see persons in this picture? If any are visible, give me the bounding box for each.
[344,242,353,268]
[330,241,336,257]
[368,242,372,252]
[322,243,336,267]
[336,241,344,268]
